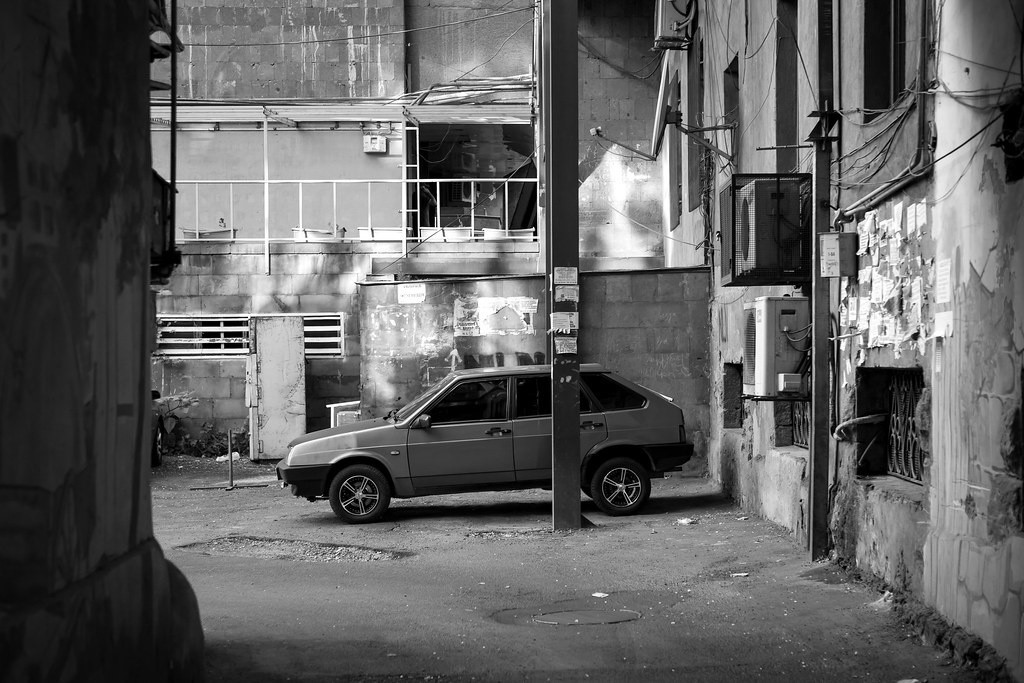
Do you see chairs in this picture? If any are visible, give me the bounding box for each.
[477,388,506,419]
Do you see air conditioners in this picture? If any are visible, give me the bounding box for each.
[736,179,800,270]
[452,152,477,173]
[452,176,477,203]
[654,0,695,49]
[742,296,813,399]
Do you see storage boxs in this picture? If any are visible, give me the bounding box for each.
[292,228,346,244]
[357,227,413,242]
[482,228,535,242]
[421,227,472,242]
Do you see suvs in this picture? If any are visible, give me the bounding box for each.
[273,362,696,525]
